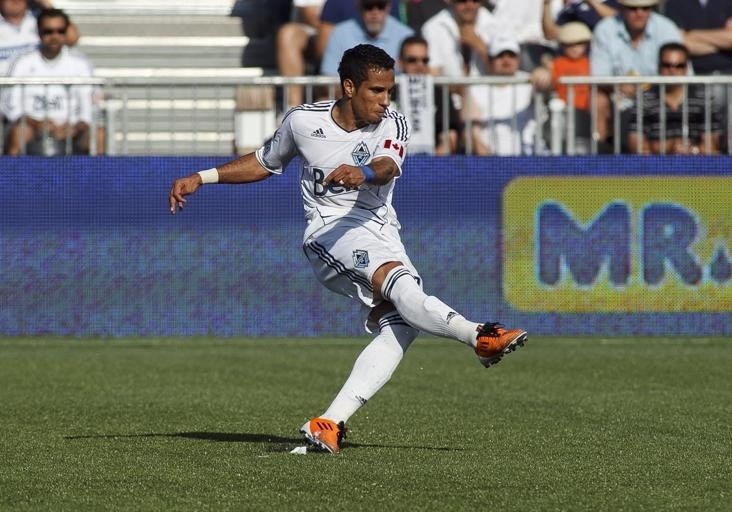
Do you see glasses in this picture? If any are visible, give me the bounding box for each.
[41,28,66,36]
[662,61,686,70]
[405,56,429,65]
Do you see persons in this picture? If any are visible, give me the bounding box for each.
[166,44,528,454]
[0,1,105,157]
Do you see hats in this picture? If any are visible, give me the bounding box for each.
[487,35,522,59]
[557,22,592,45]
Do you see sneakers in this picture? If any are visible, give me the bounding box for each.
[470,321,530,369]
[298,416,344,457]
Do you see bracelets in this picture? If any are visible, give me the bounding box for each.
[360,165,376,181]
[197,168,220,184]
